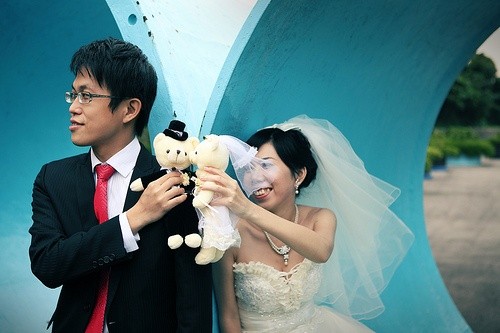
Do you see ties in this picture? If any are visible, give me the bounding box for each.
[85,163,116,332]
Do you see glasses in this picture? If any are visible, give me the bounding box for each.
[65,92,130,104]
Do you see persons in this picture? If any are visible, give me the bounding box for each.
[212,128,375,333]
[29,36,212,333]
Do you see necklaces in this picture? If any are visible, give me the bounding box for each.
[263,204,299,266]
